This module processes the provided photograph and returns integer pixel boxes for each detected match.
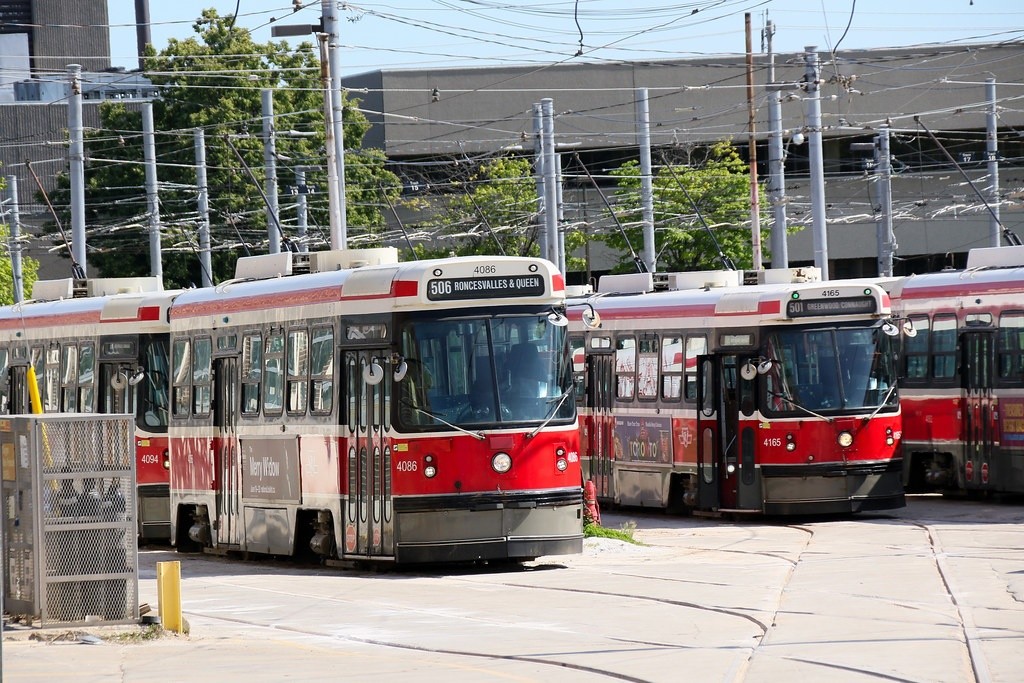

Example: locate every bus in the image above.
[555,263,918,526]
[2,276,188,544]
[156,246,589,575]
[877,244,1022,500]
